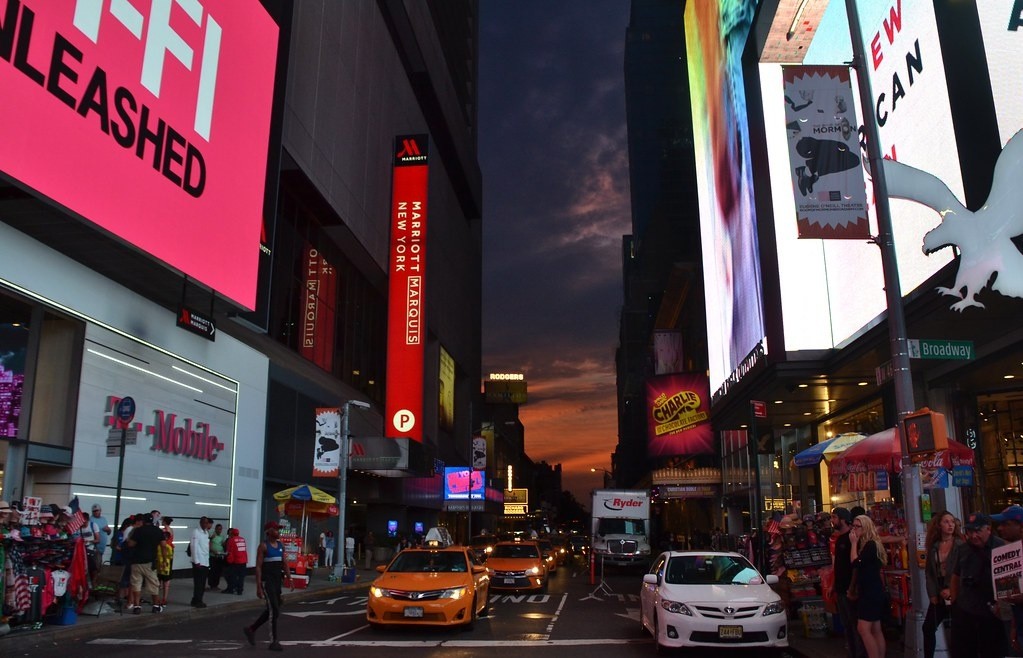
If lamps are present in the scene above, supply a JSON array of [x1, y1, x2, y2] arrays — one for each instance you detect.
[[992, 404, 996, 412], [980, 409, 985, 415], [983, 416, 988, 422]]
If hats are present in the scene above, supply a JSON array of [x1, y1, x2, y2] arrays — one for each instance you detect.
[[92, 504, 101, 512], [264, 521, 284, 531], [0, 494, 76, 569], [142, 514, 154, 523], [962, 512, 988, 530], [769, 506, 835, 576], [988, 505, 1023, 523], [231, 529, 239, 535]]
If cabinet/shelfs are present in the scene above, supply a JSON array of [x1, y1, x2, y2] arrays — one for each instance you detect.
[[770, 546, 832, 620]]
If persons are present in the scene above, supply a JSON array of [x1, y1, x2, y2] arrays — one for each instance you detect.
[[243, 521, 294, 650], [0, 500, 174, 615], [188, 516, 247, 608], [832, 504, 888, 658], [318, 528, 463, 572], [922, 504, 1023, 658]]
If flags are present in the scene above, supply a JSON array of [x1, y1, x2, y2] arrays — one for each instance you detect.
[[9, 544, 31, 612], [63, 498, 85, 534]]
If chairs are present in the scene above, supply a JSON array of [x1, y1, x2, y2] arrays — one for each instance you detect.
[[722, 563, 743, 583], [82, 560, 127, 619]]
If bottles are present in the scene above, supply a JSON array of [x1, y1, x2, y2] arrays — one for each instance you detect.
[[987, 601, 1005, 619], [943, 591, 952, 612], [872, 501, 907, 537], [893, 539, 909, 569], [289, 579, 294, 595], [888, 573, 913, 617], [1011, 640, 1016, 656]]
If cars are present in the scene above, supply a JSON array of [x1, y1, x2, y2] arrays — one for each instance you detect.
[[464, 534, 499, 559], [483, 531, 549, 594], [538, 535, 592, 574], [368, 528, 491, 630], [640, 549, 788, 657]]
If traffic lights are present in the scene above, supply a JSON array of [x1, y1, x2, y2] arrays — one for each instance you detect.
[[899, 410, 947, 461]]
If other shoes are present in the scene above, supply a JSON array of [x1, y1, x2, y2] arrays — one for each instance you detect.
[[133, 606, 142, 614], [211, 586, 220, 591], [193, 600, 206, 608], [270, 642, 282, 650], [151, 605, 162, 613], [243, 627, 255, 645], [222, 588, 234, 594]]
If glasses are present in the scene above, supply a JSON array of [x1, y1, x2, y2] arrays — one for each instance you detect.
[[851, 524, 861, 529]]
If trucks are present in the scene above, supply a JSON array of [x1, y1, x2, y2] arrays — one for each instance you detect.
[[591, 489, 652, 572]]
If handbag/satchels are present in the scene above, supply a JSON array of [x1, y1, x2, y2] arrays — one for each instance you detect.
[[186, 543, 192, 557]]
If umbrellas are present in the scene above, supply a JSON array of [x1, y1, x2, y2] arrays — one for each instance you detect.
[[789, 426, 975, 505], [273, 485, 339, 549]]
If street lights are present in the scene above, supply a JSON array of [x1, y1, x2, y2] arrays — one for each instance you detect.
[[469, 420, 516, 544], [334, 400, 372, 578]]
